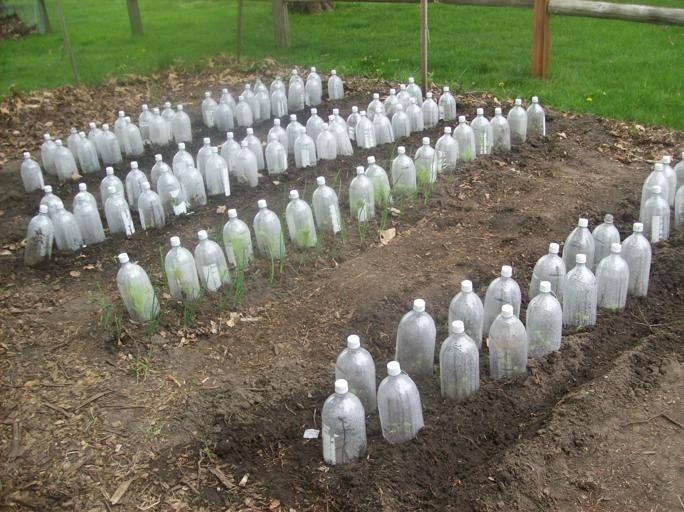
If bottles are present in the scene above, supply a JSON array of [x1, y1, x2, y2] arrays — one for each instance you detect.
[[392, 146, 416, 195], [222, 209, 253, 269], [349, 166, 375, 222], [674, 185, 684, 231], [526, 97, 545, 137], [20, 121, 104, 266], [594, 243, 629, 309], [219, 121, 283, 197], [414, 137, 437, 184], [490, 108, 511, 155], [388, 120, 431, 141], [193, 230, 230, 293], [469, 108, 491, 156], [525, 281, 562, 357], [507, 99, 527, 145], [488, 304, 527, 380], [640, 185, 670, 243], [660, 156, 677, 207], [528, 242, 566, 301], [591, 214, 620, 275], [104, 114, 162, 239], [253, 199, 285, 260], [438, 320, 479, 401], [395, 299, 436, 380], [164, 236, 200, 301], [116, 253, 160, 323], [483, 265, 521, 336], [349, 123, 388, 156], [116, 66, 457, 122], [673, 152, 684, 189], [435, 127, 457, 174], [447, 279, 484, 351], [162, 122, 221, 219], [334, 334, 377, 416], [620, 222, 652, 297], [320, 378, 367, 466], [562, 218, 594, 275], [364, 156, 392, 208], [562, 254, 597, 327], [283, 123, 348, 172], [312, 176, 341, 235], [641, 163, 670, 211], [452, 116, 475, 162], [285, 190, 316, 249], [376, 361, 424, 444]]
